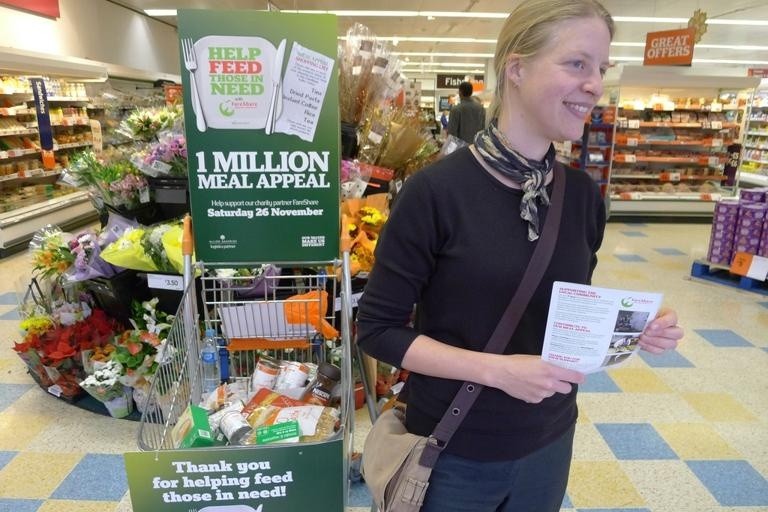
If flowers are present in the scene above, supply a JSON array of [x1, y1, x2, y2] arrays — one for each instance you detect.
[[27, 210, 183, 296], [56, 99, 182, 209], [342, 22, 444, 405], [15, 296, 182, 425]]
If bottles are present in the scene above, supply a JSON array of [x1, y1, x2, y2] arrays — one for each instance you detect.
[[198, 329, 230, 395], [307, 362, 342, 406], [746, 148, 760, 160]]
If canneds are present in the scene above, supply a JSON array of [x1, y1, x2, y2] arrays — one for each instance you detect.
[[219, 410, 253, 444], [250, 354, 318, 393]]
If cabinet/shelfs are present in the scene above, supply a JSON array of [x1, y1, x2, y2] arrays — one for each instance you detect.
[[0, 93, 162, 211], [565, 67, 768, 224]]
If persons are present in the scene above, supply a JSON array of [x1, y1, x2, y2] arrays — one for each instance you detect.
[[444, 82, 487, 143], [356, 1, 684, 512]]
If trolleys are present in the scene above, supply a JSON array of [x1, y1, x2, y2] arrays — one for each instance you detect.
[[130, 214, 384, 511]]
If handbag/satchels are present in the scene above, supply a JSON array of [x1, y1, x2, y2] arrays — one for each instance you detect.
[[358, 408, 442, 512]]
